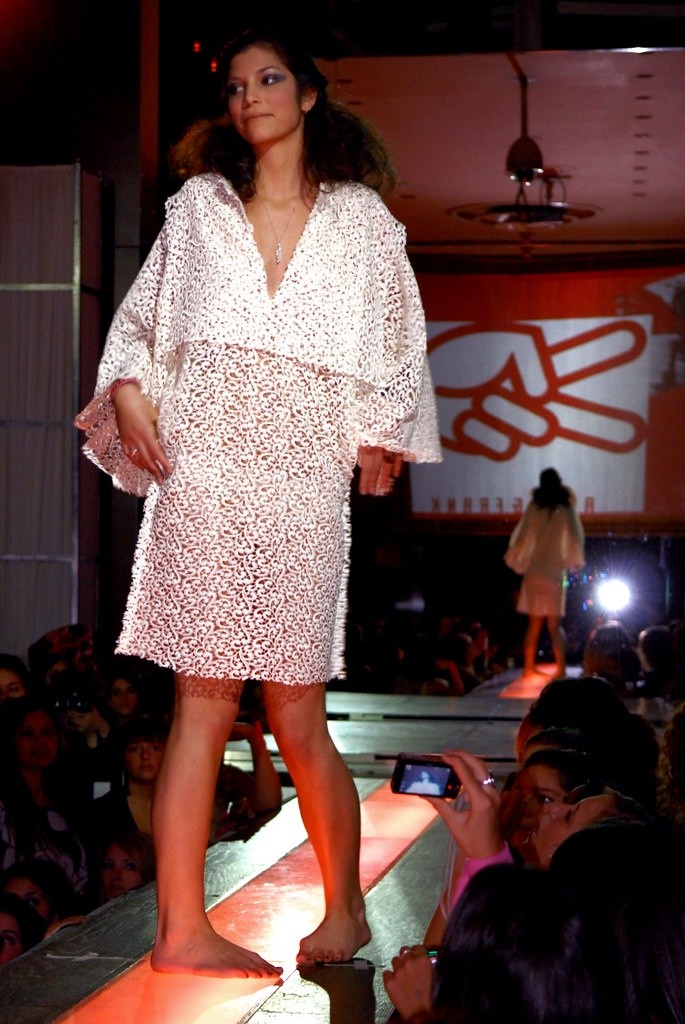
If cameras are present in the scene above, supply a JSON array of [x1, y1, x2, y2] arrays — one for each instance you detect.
[[428, 950, 440, 965], [389, 752, 462, 800]]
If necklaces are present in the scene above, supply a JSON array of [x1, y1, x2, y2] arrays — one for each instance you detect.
[[260, 198, 297, 264]]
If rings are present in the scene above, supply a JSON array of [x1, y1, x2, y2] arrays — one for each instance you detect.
[[481, 778, 495, 785], [132, 449, 137, 457], [402, 949, 413, 957]]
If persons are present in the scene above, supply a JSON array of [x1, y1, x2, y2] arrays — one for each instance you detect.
[[72, 34, 440, 978], [346, 604, 502, 700], [383, 611, 685, 1024], [504, 468, 584, 682], [406, 770, 440, 794], [0, 623, 283, 966]]
